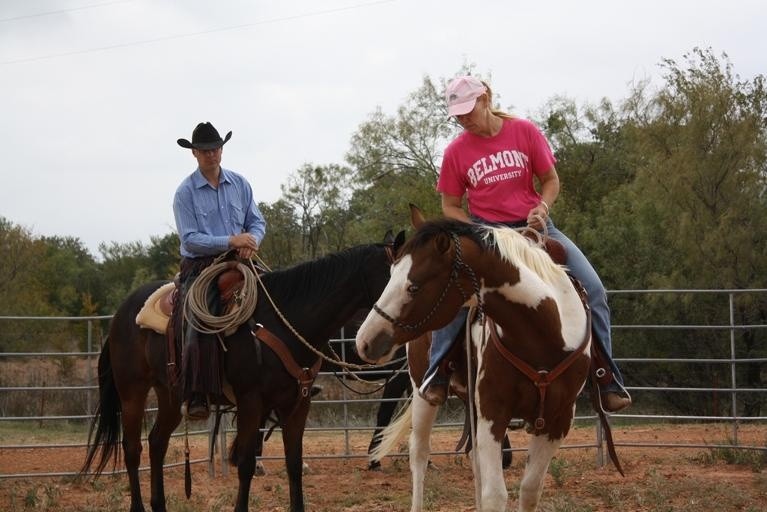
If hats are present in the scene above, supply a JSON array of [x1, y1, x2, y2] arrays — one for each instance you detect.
[[445, 76, 487, 121], [177, 122, 232, 151]]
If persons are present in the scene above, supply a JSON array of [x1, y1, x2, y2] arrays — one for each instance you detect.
[[173, 122, 324, 418], [422, 76, 631, 411]]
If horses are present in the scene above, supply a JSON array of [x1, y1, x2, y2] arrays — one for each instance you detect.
[[355, 203, 593, 512], [79, 230, 406, 512], [228, 309, 511, 476]]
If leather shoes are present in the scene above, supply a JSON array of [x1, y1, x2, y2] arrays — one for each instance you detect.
[[185, 399, 208, 421], [593, 392, 630, 413], [422, 380, 446, 405]]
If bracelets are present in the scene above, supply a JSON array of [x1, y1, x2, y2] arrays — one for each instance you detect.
[[540, 201, 549, 215]]
